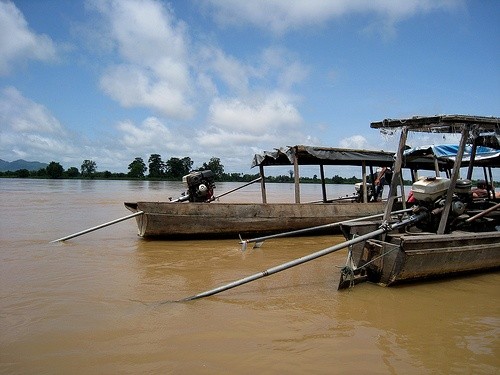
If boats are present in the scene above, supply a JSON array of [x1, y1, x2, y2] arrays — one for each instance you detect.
[[123, 141, 461, 241], [337, 112, 500, 291]]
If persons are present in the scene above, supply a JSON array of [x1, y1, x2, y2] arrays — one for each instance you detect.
[[374, 166, 387, 202]]
